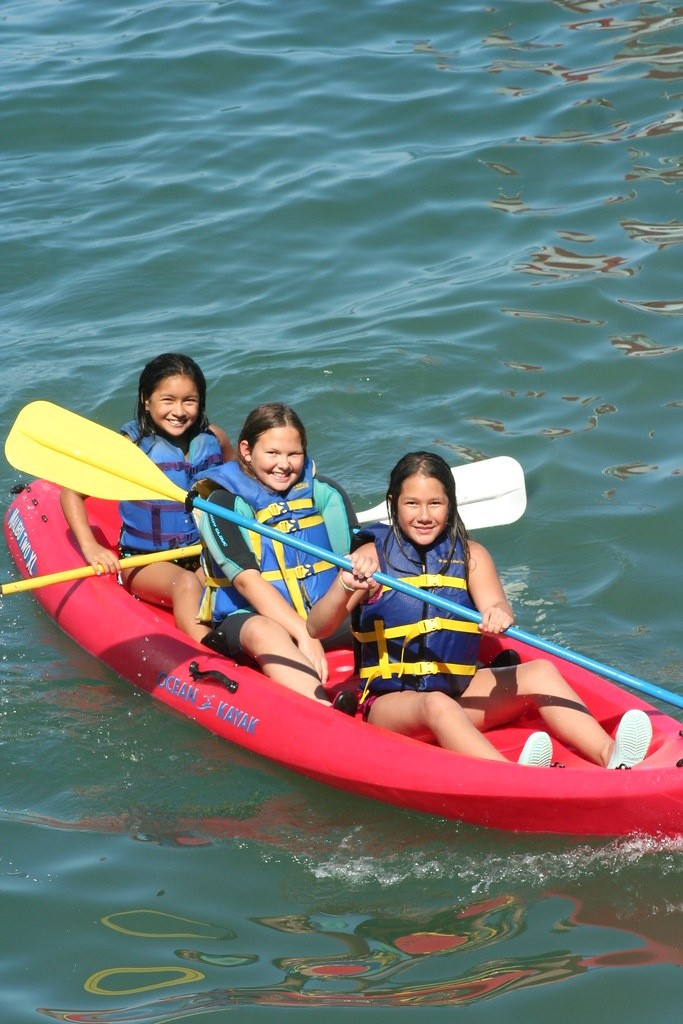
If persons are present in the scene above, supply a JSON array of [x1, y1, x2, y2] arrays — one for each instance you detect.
[[306, 450, 652, 769], [184, 403, 378, 718], [60, 354, 237, 645]]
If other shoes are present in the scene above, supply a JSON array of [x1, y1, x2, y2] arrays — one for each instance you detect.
[[606, 709, 653, 769], [518, 731, 554, 768], [329, 690, 358, 718], [485, 649, 521, 668]]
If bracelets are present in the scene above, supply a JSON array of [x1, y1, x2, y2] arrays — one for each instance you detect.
[[340, 574, 356, 592]]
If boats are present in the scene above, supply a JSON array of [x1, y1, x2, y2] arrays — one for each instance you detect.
[[4, 477, 682, 838]]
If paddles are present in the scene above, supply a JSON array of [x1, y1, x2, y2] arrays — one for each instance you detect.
[[1, 453, 530, 599], [1, 395, 683, 712]]
